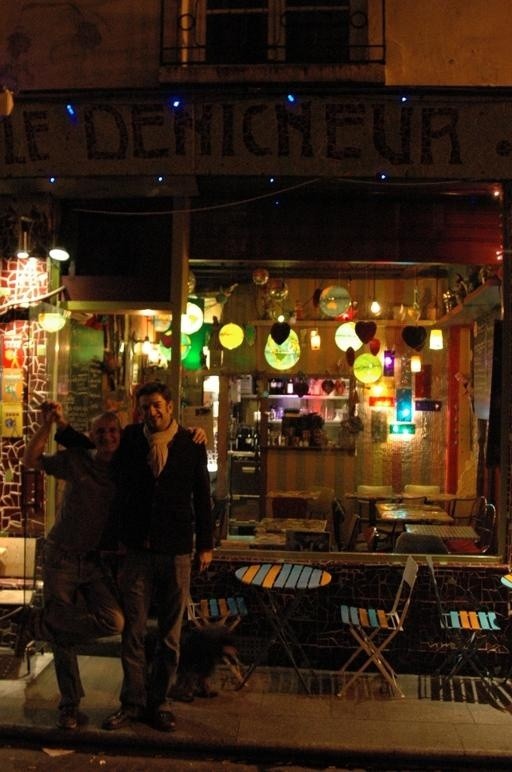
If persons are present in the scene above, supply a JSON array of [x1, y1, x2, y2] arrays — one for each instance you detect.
[[40, 380, 216, 730], [12, 403, 208, 730]]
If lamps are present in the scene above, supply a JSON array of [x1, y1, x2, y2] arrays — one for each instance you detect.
[[16, 241, 445, 441]]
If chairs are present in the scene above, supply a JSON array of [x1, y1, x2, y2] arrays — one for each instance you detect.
[[0, 537, 37, 658], [227, 485, 497, 554], [188, 597, 264, 687], [425, 555, 501, 703], [336, 554, 419, 699]]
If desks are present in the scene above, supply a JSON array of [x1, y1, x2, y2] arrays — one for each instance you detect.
[[501, 572, 512, 596], [234, 564, 333, 695]]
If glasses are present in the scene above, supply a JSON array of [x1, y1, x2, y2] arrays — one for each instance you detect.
[[96, 428, 119, 434]]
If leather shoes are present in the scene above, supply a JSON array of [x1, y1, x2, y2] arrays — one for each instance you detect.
[[56, 705, 78, 728], [102, 710, 133, 730], [148, 706, 177, 732], [15, 609, 32, 658]]
